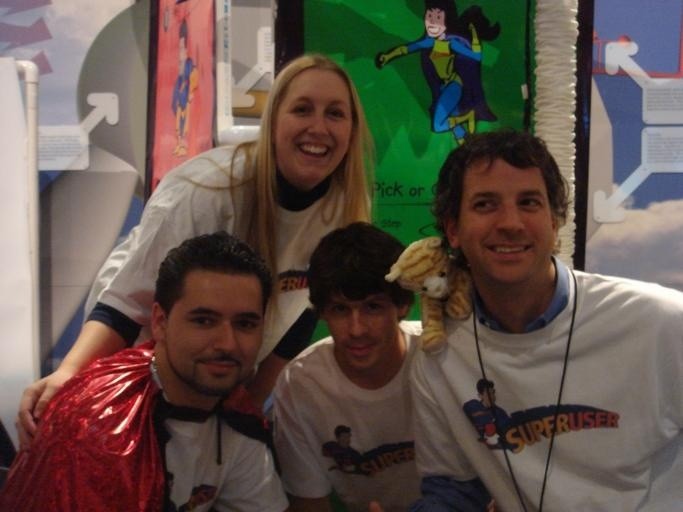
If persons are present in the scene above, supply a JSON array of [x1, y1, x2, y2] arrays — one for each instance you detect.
[[376, 2, 503, 145], [414, 130, 683, 508], [272, 223, 424, 507], [468, 378, 520, 454], [15, 54, 370, 446], [1, 232, 290, 508], [315, 418, 372, 479]]
